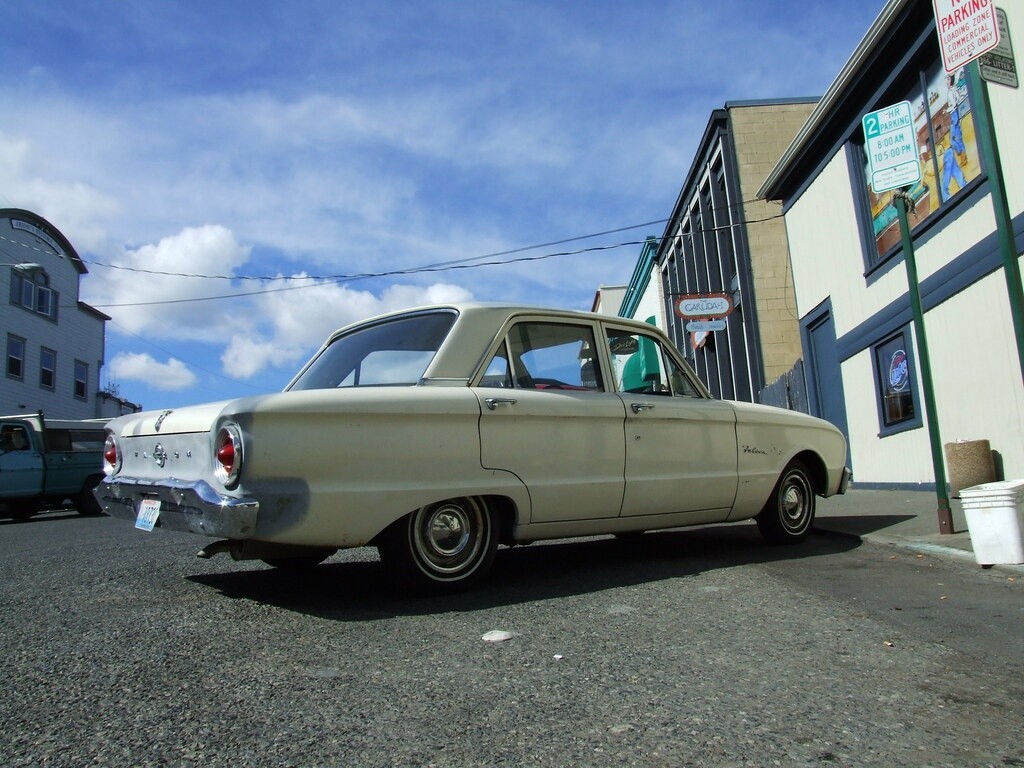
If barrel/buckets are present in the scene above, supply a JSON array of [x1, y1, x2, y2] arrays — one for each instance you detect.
[[960, 478, 1024, 565]]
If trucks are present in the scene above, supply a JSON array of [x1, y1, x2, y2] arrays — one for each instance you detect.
[[0, 408, 107, 516]]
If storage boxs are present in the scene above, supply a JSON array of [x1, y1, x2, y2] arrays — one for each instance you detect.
[[959, 479, 1024, 565]]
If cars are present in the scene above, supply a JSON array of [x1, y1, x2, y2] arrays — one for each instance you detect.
[[91, 303, 856, 595]]
